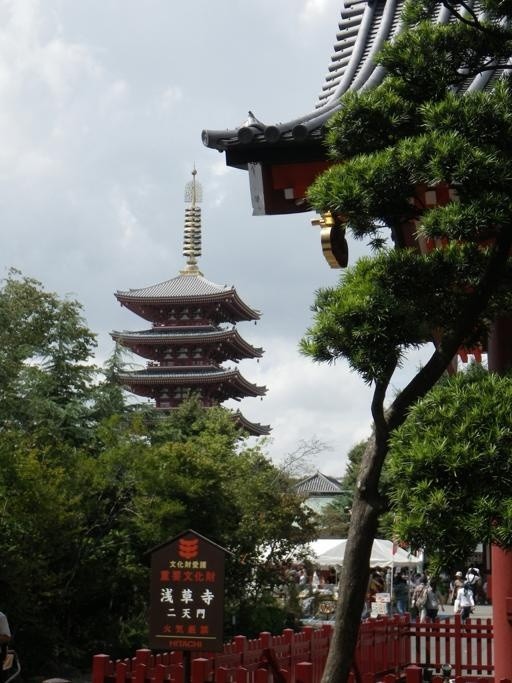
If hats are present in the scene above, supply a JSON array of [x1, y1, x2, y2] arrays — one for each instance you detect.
[[454, 570, 463, 577]]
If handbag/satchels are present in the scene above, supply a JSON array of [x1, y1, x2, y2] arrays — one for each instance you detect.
[[415, 587, 429, 609]]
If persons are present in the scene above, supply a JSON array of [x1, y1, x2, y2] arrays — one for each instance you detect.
[[279, 560, 493, 640], [0, 611, 12, 654]]
